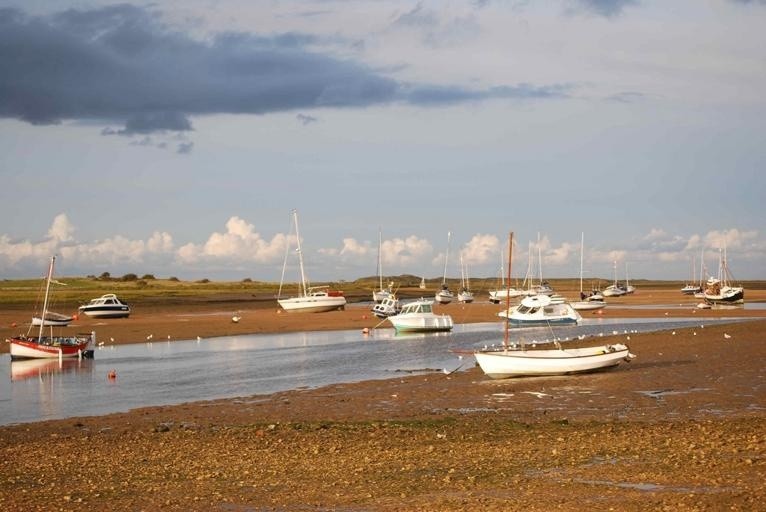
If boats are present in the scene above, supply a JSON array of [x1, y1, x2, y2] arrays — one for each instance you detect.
[[78, 294, 132, 319], [32, 316, 75, 327]]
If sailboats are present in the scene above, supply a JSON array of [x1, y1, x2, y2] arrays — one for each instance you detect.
[[369, 227, 636, 334], [682, 241, 746, 306], [472, 231, 638, 379], [6, 255, 97, 362], [277, 209, 346, 315]]
